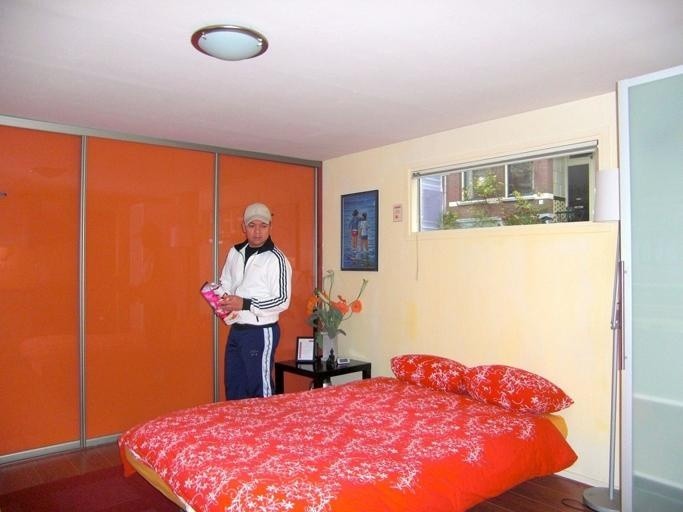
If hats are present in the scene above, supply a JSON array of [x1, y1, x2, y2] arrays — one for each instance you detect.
[[243, 203, 271, 226]]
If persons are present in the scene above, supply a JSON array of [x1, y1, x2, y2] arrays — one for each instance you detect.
[[357, 213, 369, 252], [348, 209, 367, 249], [209, 202, 292, 400]]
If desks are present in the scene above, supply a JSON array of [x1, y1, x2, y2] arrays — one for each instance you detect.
[[273, 355, 372, 394]]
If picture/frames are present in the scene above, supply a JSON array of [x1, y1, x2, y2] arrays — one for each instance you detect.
[[340, 189, 379, 272], [295, 336, 315, 364]]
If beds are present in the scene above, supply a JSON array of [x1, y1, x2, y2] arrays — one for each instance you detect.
[[117, 376, 568, 512]]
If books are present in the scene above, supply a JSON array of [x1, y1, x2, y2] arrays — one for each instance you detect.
[[199, 280, 241, 326]]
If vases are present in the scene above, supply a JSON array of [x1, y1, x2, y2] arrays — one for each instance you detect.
[[320, 330, 335, 362]]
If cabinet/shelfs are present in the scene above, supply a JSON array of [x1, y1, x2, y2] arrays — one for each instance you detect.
[[218, 144, 322, 399], [2, 114, 82, 462], [83, 126, 217, 464]]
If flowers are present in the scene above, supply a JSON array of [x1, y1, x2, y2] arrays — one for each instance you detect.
[[305, 269, 369, 348]]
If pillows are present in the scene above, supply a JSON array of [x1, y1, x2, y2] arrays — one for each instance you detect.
[[390, 353, 470, 395], [452, 363, 575, 415]]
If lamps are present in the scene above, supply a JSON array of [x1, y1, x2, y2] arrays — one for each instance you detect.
[[580, 166, 625, 511], [191, 23, 269, 62]]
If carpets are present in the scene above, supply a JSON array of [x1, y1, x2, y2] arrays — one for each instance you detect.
[[0, 462, 184, 512]]
